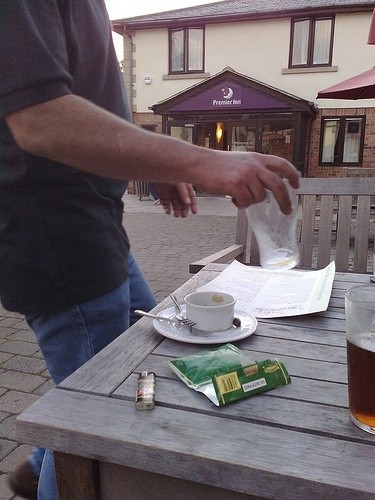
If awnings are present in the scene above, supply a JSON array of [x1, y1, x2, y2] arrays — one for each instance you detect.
[[316, 66, 375, 100]]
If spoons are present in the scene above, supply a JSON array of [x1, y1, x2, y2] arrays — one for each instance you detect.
[[170, 294, 186, 321]]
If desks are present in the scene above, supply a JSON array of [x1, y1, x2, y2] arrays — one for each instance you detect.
[[16, 263, 375, 500]]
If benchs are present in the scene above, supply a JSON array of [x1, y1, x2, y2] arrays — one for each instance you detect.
[[189, 176, 375, 273]]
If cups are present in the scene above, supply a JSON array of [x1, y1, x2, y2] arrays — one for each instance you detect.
[[184, 291, 237, 332], [245, 179, 300, 270], [344, 284, 375, 436]]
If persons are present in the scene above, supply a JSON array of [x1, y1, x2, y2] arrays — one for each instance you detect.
[[0, 0, 300, 500]]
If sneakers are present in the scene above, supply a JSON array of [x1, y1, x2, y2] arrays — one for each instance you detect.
[[9, 461, 40, 500]]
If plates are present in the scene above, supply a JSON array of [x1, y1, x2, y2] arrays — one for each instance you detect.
[[153, 305, 257, 344]]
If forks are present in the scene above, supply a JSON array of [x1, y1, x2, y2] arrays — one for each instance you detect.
[[134, 310, 197, 326]]
